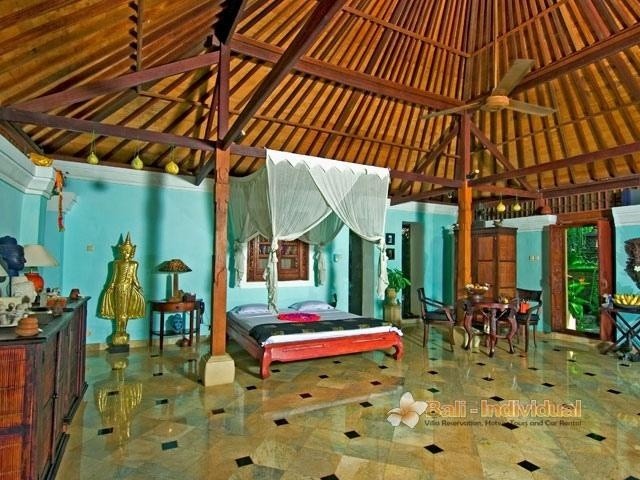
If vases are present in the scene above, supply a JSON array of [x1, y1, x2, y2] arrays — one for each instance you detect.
[[469, 293, 483, 303]]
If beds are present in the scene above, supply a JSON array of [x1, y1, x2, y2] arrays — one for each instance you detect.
[[465, 298, 519, 357], [227, 300, 404, 379]]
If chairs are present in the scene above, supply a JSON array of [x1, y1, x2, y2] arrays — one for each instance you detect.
[[496, 288, 543, 351], [419, 288, 457, 352]]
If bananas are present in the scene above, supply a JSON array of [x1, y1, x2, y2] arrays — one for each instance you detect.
[[612, 294, 640, 305]]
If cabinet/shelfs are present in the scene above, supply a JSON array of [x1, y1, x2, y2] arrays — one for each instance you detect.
[[150, 300, 201, 348], [0, 312, 70, 480], [454, 228, 518, 327], [64, 296, 89, 427]]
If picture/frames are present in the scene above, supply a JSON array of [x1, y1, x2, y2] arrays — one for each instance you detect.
[[386, 249, 394, 260], [386, 233, 395, 245]]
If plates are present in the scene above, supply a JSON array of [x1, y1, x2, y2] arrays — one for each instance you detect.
[[615, 304, 640, 311], [469, 289, 487, 299]]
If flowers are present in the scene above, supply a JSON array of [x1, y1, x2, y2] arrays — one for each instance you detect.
[[465, 283, 489, 295]]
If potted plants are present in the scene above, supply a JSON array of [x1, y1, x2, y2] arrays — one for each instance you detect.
[[385, 269, 412, 301]]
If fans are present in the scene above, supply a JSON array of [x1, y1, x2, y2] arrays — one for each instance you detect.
[[420, 0, 557, 120]]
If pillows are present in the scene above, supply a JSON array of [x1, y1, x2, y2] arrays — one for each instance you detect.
[[289, 300, 334, 311], [159, 260, 192, 302], [231, 303, 272, 314]]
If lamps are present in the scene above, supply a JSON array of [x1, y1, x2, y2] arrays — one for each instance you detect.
[[24, 244, 58, 291]]
